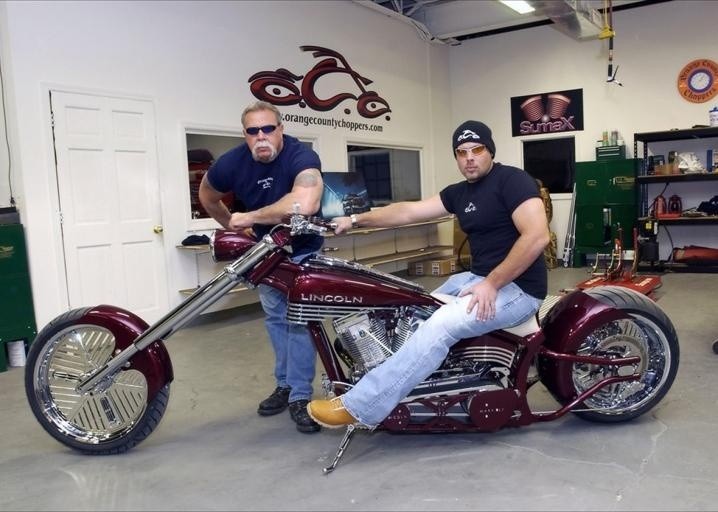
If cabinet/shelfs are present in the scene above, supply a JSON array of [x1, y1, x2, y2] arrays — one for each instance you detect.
[[0, 223, 38, 372], [633, 127, 718, 273], [572, 155, 645, 267], [175, 216, 457, 297]]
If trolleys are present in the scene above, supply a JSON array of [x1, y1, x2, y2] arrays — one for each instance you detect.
[[567, 216, 665, 305]]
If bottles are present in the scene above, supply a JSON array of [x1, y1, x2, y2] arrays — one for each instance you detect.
[[654, 195, 682, 216], [602, 127, 618, 146]]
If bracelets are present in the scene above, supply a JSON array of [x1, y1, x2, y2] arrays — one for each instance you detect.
[[350, 214, 359, 229]]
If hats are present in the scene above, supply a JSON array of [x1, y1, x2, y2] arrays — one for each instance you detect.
[[451, 119, 496, 160]]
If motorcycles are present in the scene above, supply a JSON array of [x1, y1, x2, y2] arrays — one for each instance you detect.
[[16, 199, 684, 479]]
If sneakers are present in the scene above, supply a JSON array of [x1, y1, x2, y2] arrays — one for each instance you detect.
[[257, 386, 291, 417], [287, 398, 321, 435], [306, 394, 373, 432]]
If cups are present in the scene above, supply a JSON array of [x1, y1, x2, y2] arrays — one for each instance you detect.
[[616, 139, 625, 146], [660, 164, 674, 175]]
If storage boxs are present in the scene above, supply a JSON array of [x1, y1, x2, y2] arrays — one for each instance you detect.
[[408, 253, 472, 276]]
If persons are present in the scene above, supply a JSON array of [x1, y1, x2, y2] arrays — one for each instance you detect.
[[307, 119, 550, 432], [199, 102, 325, 433]]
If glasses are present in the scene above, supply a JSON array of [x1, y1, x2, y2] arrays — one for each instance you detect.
[[245, 124, 279, 134], [453, 144, 487, 157]]
